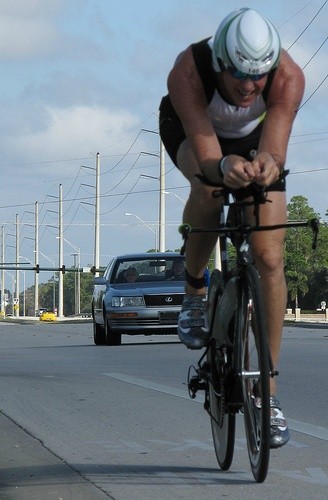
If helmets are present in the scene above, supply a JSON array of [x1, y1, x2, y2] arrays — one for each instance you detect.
[[212, 7, 280, 75]]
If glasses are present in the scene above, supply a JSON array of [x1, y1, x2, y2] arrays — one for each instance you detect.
[[228, 67, 267, 81]]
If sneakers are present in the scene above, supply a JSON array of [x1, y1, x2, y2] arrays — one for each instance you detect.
[[177, 295, 210, 348], [252, 384, 290, 448]]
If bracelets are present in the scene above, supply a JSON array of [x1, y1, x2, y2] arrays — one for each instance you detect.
[[217, 155, 227, 178]]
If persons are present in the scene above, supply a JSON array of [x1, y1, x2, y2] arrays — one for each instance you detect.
[[124, 267, 139, 282], [166, 259, 187, 280], [159, 7, 306, 449]]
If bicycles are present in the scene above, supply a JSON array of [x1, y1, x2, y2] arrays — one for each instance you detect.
[[179, 167, 319, 484]]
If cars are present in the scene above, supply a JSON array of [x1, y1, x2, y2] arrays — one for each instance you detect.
[[38, 308, 47, 317], [39, 312, 56, 321], [91, 252, 210, 346]]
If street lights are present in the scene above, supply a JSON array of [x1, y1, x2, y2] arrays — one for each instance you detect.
[[55, 236, 81, 314], [124, 213, 158, 273], [32, 250, 55, 314]]
